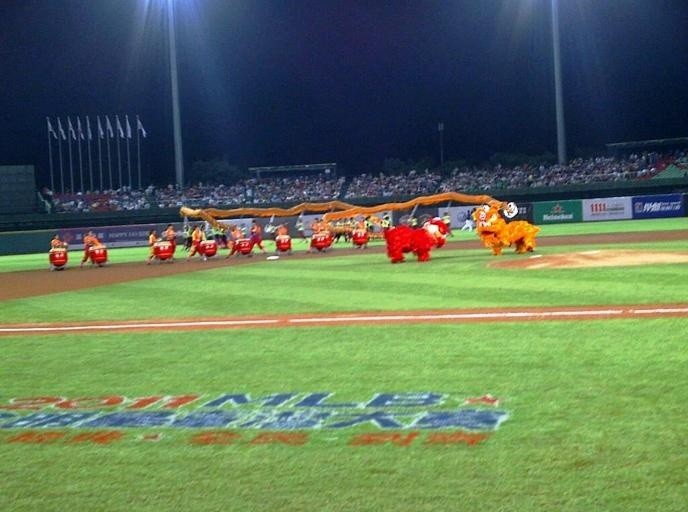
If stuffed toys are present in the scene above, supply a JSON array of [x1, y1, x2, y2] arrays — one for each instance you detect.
[[472, 205, 540, 256], [383, 217, 451, 264]]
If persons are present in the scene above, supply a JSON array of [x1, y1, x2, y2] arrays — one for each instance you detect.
[[45, 148, 688, 216], [398, 207, 476, 238], [50, 231, 103, 267], [148, 212, 390, 262]]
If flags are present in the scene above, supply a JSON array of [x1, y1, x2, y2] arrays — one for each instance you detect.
[[86, 116, 92, 140], [138, 119, 146, 138], [97, 116, 104, 139], [77, 117, 84, 140], [116, 114, 124, 138], [126, 118, 131, 138], [106, 115, 113, 138], [58, 119, 66, 140], [48, 120, 57, 139], [67, 116, 76, 141]]
